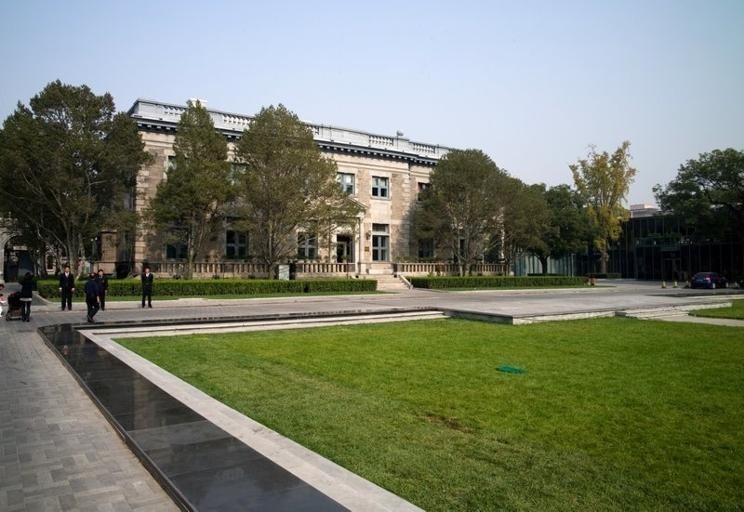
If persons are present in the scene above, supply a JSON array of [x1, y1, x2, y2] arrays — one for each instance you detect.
[[83, 271, 101, 323], [19, 272, 33, 322], [0, 281, 4, 291], [0, 294, 7, 320], [140, 266, 153, 309], [55, 265, 74, 311], [94, 268, 108, 311]]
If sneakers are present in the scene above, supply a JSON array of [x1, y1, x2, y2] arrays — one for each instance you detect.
[[87, 314, 93, 322]]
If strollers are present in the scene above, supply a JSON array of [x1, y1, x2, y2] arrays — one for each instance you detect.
[[6, 292, 23, 321]]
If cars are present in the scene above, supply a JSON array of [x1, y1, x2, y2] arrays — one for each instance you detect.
[[692, 272, 726, 289]]
